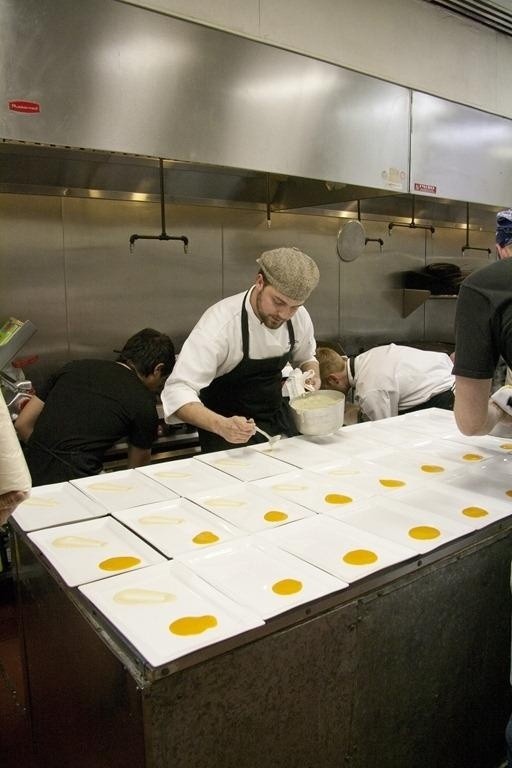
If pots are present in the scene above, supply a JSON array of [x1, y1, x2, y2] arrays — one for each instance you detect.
[[289, 390, 344, 436]]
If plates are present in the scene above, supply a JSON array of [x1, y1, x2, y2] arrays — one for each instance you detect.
[[112, 497, 248, 560], [248, 430, 344, 470], [9, 482, 109, 534], [184, 481, 319, 535], [136, 453, 249, 496], [77, 558, 267, 667], [68, 468, 179, 512], [253, 513, 420, 585], [250, 466, 367, 515], [195, 439, 302, 483], [305, 406, 512, 531], [325, 494, 476, 555], [26, 514, 164, 588], [175, 534, 349, 622]]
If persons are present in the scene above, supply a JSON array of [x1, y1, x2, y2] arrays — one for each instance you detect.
[[160, 245, 323, 454], [314, 340, 457, 424], [449, 207, 511, 437], [12, 327, 178, 489]]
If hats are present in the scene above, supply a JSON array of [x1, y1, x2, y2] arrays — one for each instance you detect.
[[256, 246, 320, 301], [495, 209, 512, 230]]
